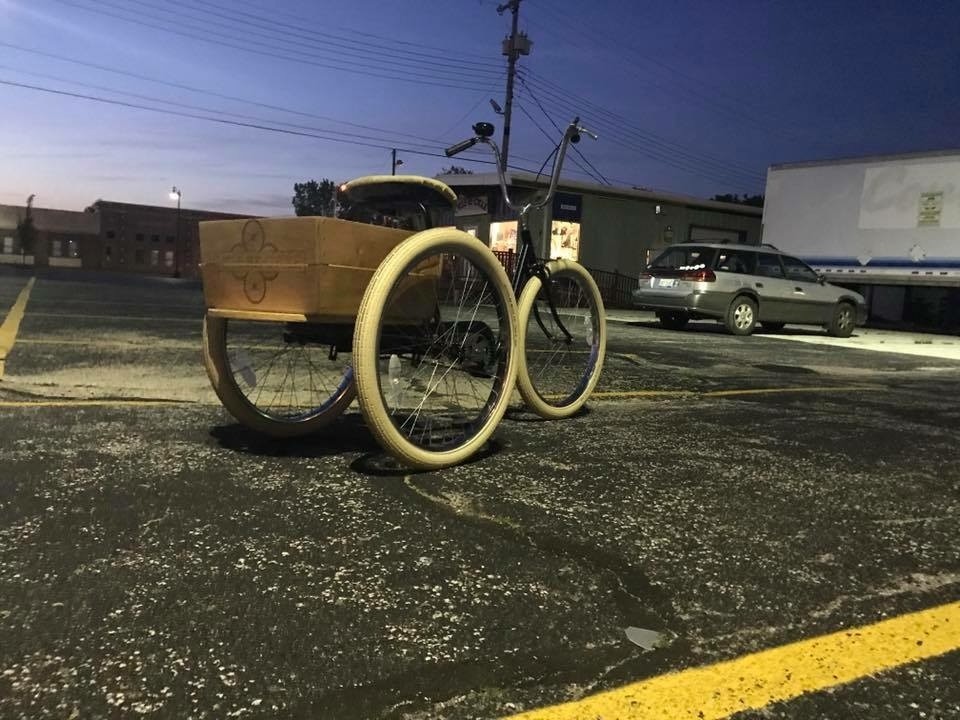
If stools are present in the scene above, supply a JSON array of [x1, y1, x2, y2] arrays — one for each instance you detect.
[[341, 175, 457, 229]]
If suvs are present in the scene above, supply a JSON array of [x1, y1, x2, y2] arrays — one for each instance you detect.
[[628, 240, 868, 338]]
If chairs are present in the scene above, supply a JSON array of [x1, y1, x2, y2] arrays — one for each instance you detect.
[[735, 263, 749, 273], [758, 265, 773, 276], [691, 253, 722, 268]]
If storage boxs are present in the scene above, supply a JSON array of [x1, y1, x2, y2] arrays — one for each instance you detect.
[[198, 218, 438, 319]]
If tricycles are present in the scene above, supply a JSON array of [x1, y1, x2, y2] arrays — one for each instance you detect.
[[197, 116, 608, 474]]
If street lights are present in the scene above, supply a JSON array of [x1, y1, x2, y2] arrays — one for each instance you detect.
[[168, 186, 182, 279], [391, 148, 404, 176], [488, 99, 514, 269]]
